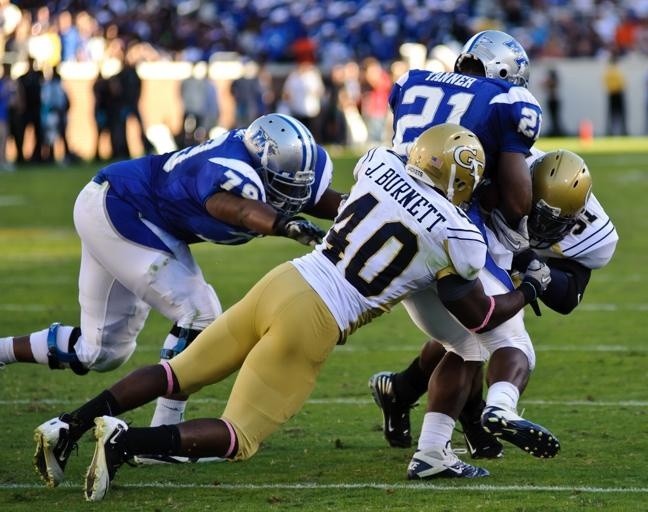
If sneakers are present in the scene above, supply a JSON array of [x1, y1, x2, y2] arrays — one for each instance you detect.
[[83, 415, 130, 501], [461, 402, 504, 461], [480, 406, 560, 458], [134, 452, 225, 465], [368, 370, 412, 447], [407, 438, 490, 480], [32, 412, 77, 488]]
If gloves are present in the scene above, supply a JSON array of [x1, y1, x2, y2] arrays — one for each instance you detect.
[[272, 213, 325, 245], [516, 258, 552, 303], [491, 209, 533, 266]]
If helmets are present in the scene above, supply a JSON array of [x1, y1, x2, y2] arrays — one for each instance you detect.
[[454, 29, 530, 88], [244, 112, 317, 216], [527, 149, 593, 250], [406, 122, 486, 205]]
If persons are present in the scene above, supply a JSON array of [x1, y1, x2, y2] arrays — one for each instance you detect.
[[32, 121, 552, 502], [368, 29, 561, 482], [0, 114, 346, 465], [1, 1, 647, 168], [369, 145, 620, 461]]
